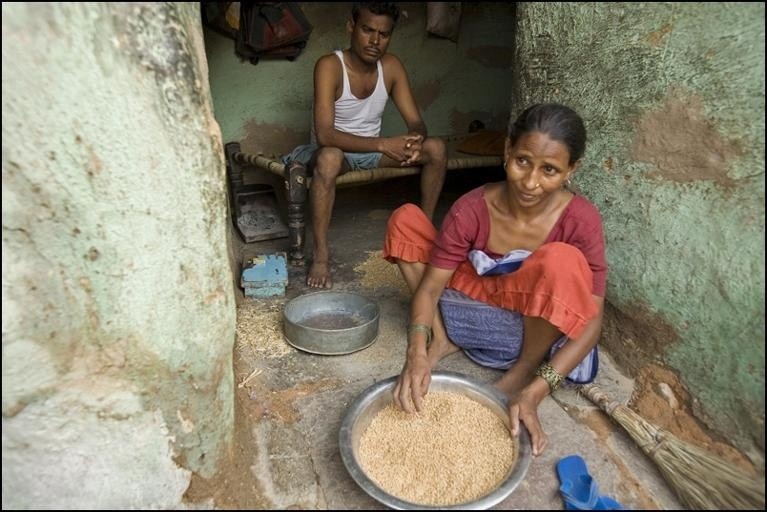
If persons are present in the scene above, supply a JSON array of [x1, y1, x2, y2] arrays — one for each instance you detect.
[[381, 103, 608, 457], [306, 2, 448, 289]]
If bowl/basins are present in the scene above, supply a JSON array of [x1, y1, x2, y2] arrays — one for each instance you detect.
[[336, 370, 536, 510]]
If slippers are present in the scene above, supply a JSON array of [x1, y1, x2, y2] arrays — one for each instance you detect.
[[555, 455, 608, 511]]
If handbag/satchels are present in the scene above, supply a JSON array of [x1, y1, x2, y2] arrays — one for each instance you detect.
[[235, 2, 314, 65], [425, 0, 462, 43]]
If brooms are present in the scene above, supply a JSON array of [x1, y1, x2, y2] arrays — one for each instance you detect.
[[576, 377, 765, 511]]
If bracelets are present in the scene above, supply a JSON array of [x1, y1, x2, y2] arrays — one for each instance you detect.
[[536, 362, 561, 395], [408, 324, 432, 350]]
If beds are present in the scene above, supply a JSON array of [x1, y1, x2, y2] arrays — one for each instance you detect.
[[224, 139, 504, 265]]
